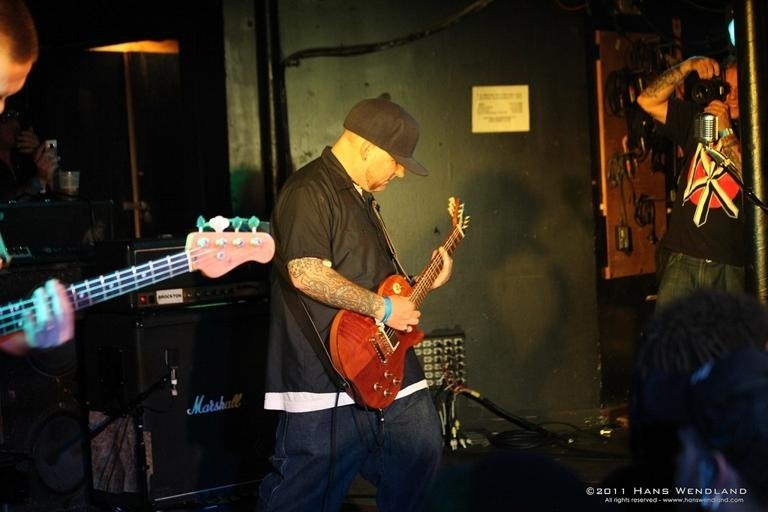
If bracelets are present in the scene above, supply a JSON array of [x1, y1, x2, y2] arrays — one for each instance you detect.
[[380, 296, 392, 323]]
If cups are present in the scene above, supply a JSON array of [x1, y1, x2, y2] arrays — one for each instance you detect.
[[57, 166, 80, 202]]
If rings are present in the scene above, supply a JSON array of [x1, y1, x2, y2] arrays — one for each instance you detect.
[[404, 326, 408, 333]]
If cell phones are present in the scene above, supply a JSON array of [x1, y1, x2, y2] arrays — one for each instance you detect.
[[45, 139, 58, 157]]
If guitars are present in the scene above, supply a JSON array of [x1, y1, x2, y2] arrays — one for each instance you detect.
[[0, 217, 275, 338], [330, 196, 471, 409]]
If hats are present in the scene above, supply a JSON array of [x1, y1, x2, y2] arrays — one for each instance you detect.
[[342, 97, 429, 176]]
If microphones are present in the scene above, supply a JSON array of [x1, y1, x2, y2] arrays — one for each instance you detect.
[[692, 111, 718, 144]]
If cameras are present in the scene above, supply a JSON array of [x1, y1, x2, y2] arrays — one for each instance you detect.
[[692, 69, 731, 106]]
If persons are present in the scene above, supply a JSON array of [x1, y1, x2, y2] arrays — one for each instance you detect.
[[0, 0, 77, 358], [586, 286, 768, 510], [640, 343, 768, 510], [257, 98, 452, 512], [0, 104, 54, 200], [634, 53, 765, 312]]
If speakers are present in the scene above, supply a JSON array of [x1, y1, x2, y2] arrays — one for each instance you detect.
[[128, 302, 267, 504], [0, 260, 134, 512]]
